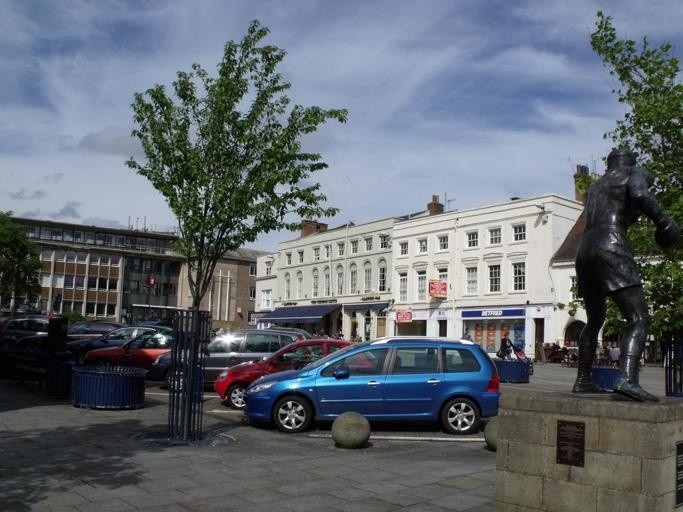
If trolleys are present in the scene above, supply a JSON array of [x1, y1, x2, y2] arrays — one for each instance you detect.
[[510, 349, 534, 376]]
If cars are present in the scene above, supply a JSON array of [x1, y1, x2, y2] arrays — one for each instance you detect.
[[243, 336, 500, 433]]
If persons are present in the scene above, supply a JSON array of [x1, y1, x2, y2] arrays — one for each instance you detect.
[[571, 148, 681, 401], [461, 328, 473, 342], [500, 331, 520, 360]]
